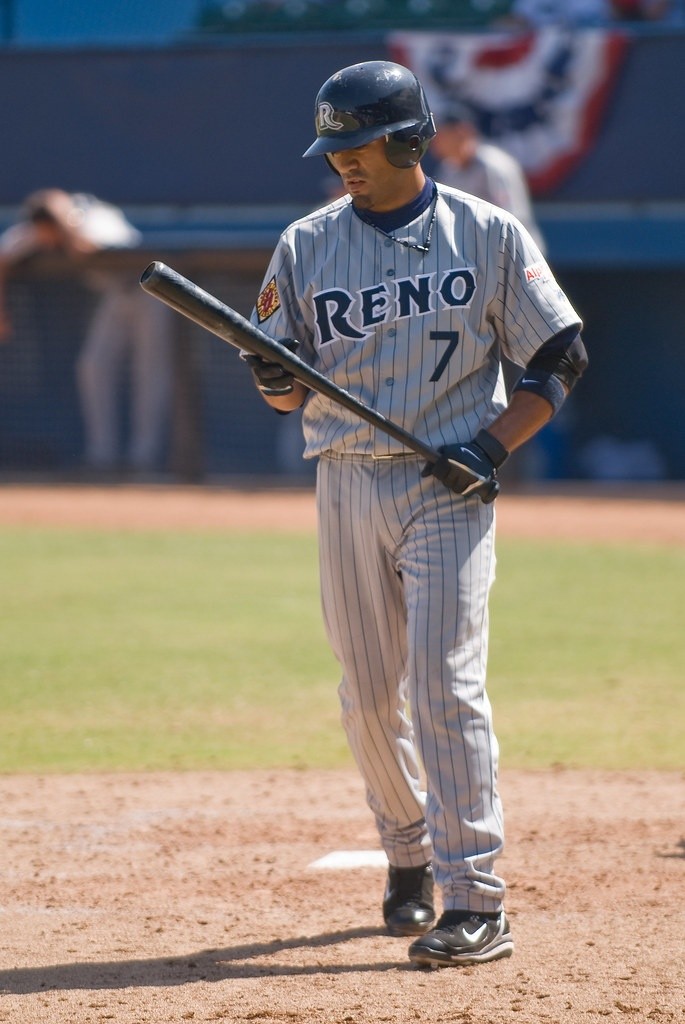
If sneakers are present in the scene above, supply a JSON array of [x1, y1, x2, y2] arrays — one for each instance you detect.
[[408, 910, 514, 969], [383, 862, 435, 935]]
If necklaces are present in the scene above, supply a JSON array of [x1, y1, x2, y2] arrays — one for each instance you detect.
[[358, 177, 440, 255]]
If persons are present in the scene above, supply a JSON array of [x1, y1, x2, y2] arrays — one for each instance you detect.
[[430, 101, 546, 257], [239, 60, 589, 966], [0, 188, 170, 480]]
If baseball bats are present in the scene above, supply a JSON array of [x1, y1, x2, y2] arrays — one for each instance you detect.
[[139, 260, 502, 506]]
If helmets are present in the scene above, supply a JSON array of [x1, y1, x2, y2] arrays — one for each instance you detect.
[[301, 60, 437, 176]]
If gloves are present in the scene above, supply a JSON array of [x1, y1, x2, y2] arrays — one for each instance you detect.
[[243, 338, 300, 396], [420, 428, 510, 498]]
